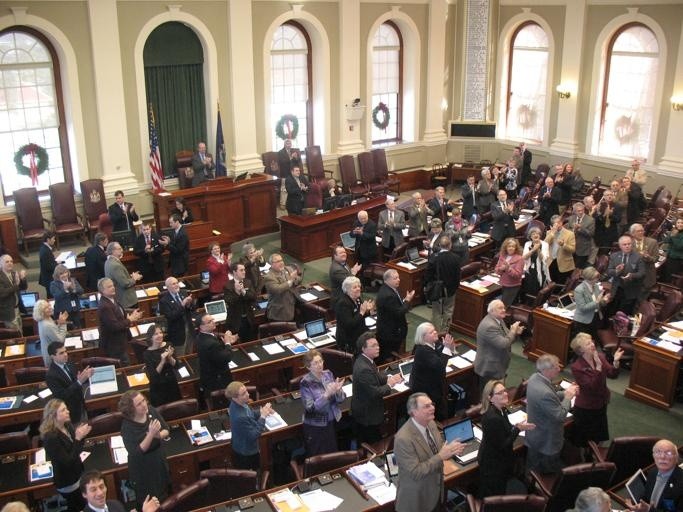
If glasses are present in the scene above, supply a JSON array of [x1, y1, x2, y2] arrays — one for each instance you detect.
[[492, 387, 510, 396]]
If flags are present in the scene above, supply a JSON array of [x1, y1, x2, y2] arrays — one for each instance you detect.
[[215, 110, 228, 177], [145, 108, 169, 193]]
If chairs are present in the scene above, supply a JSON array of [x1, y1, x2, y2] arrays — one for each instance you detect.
[[338, 149, 401, 197], [50, 183, 87, 251], [464, 436, 670, 512], [177, 150, 199, 189], [15, 187, 54, 256], [432, 164, 448, 188], [304, 146, 337, 191], [81, 179, 109, 244]]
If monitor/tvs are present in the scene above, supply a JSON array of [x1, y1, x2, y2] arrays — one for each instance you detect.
[[323, 194, 353, 212], [234, 172, 248, 181], [112, 227, 175, 251]]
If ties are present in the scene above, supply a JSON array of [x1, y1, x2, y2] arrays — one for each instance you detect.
[[63, 363, 73, 380], [114, 299, 125, 320], [389, 210, 393, 225], [425, 427, 439, 456], [622, 253, 628, 271]]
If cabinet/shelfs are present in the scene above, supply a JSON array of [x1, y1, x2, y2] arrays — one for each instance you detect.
[[2, 213, 21, 263]]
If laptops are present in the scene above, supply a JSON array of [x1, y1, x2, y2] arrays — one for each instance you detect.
[[19, 292, 39, 318], [407, 246, 428, 265], [398, 358, 415, 382], [444, 418, 482, 465], [204, 299, 228, 323], [304, 318, 336, 348], [385, 450, 399, 488], [559, 293, 576, 312], [340, 231, 356, 252], [626, 469, 648, 504], [200, 271, 210, 284], [88, 364, 119, 396]]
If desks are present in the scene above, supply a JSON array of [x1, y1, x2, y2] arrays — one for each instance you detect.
[[330, 199, 681, 414], [278, 192, 399, 261], [1, 221, 526, 512], [449, 163, 502, 187], [155, 171, 280, 239]]
